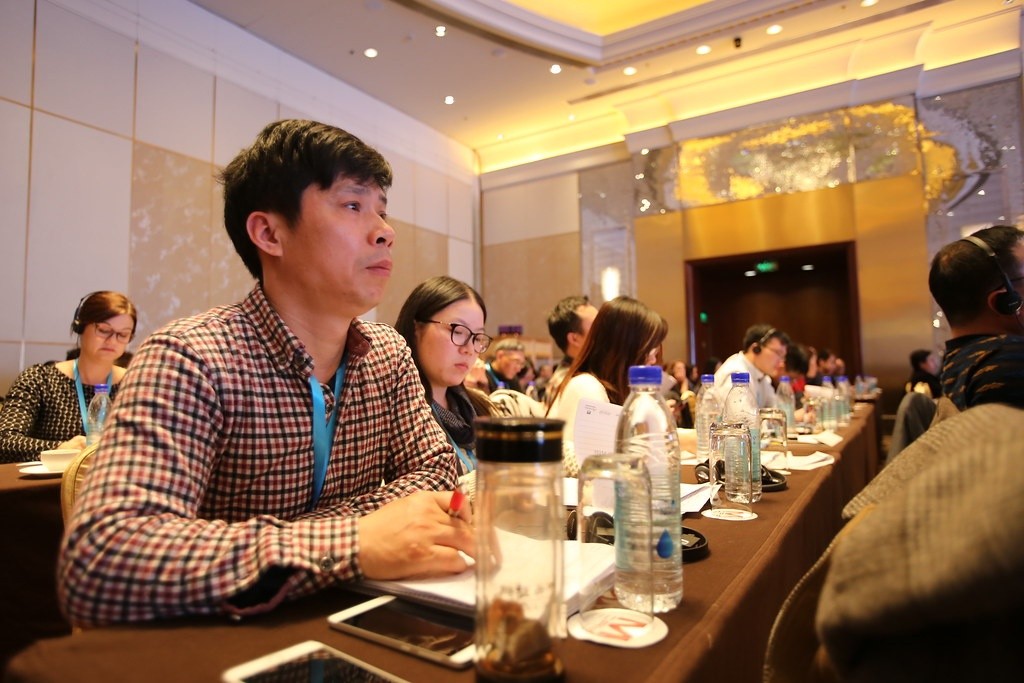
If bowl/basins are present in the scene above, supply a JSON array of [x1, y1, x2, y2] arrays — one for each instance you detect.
[[40, 449, 82, 472]]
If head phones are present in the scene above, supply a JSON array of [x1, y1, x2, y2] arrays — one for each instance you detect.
[[695, 459, 787, 491], [959, 236, 1021, 315], [753, 328, 776, 353], [73, 292, 97, 334], [567, 509, 708, 561]]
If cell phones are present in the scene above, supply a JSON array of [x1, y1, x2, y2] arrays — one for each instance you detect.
[[328, 595, 492, 668], [222, 639, 412, 683]]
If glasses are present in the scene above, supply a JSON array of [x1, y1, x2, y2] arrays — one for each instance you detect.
[[91, 322, 135, 344], [415, 319, 494, 354]]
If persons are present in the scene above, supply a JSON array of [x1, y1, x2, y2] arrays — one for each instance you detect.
[[462, 338, 559, 403], [661, 357, 722, 430], [928, 225, 1024, 409], [546, 294, 599, 408], [546, 295, 698, 481], [692, 324, 807, 433], [768, 344, 846, 410], [909, 350, 942, 398], [394, 276, 501, 513], [51, 117, 497, 630], [0, 292, 137, 464]]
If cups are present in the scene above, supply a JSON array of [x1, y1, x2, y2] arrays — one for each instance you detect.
[[708, 421, 753, 519], [576, 456, 656, 630], [803, 396, 824, 432], [759, 408, 788, 470]]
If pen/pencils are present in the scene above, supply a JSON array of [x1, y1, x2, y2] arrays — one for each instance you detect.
[[448, 482, 465, 515]]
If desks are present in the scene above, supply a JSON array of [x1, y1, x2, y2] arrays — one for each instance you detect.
[[3, 403, 879, 683], [0, 461, 69, 683]]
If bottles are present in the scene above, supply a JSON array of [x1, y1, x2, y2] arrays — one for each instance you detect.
[[696, 374, 724, 464], [496, 382, 506, 389], [774, 375, 795, 433], [615, 363, 684, 613], [526, 381, 539, 402], [838, 374, 878, 428], [469, 416, 569, 683], [725, 372, 762, 502], [821, 376, 838, 431], [85, 383, 113, 449]]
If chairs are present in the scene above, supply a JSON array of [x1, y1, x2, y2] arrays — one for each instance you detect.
[[886, 391, 936, 464]]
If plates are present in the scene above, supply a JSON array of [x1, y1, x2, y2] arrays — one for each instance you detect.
[[19, 465, 64, 476]]
